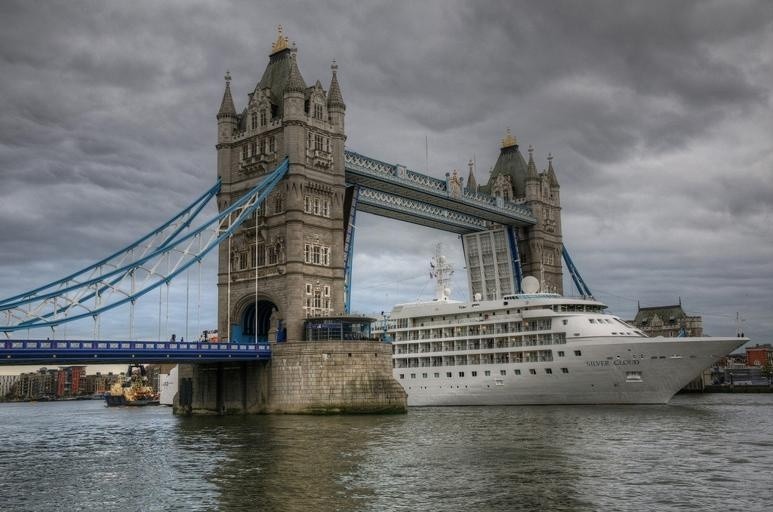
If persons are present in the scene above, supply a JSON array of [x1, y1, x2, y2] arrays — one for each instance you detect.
[[170, 334, 176, 341]]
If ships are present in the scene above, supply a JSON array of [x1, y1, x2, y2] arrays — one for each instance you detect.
[[103, 364, 160, 406], [159, 240, 751, 406]]
